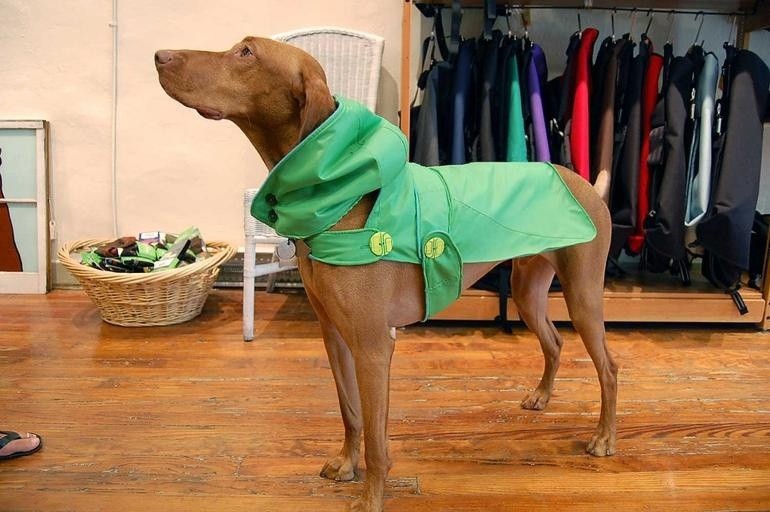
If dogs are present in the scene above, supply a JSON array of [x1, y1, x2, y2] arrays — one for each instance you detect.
[[154, 36, 619, 512]]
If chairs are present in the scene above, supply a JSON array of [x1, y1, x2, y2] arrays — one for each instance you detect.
[[238, 27, 392, 342]]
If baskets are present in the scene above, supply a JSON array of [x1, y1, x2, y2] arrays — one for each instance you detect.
[[56, 237, 236, 328]]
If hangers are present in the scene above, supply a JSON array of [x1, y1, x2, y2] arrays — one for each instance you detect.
[[443, 4, 742, 59]]
